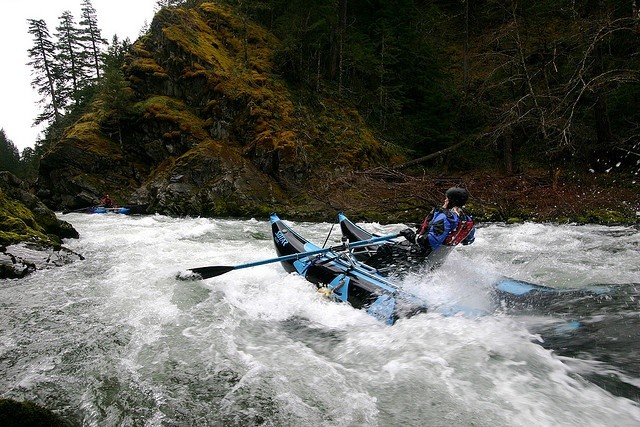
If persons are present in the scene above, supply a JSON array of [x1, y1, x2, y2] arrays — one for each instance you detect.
[[100, 195, 113, 208], [389, 186, 475, 267]]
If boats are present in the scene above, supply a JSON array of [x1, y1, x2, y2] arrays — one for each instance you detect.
[[269, 212, 640, 351], [88, 205, 131, 214]]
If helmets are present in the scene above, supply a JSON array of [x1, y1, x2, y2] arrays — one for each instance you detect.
[[446, 187, 468, 209]]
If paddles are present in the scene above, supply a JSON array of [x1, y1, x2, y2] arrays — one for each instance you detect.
[[171, 234, 402, 282], [62, 203, 103, 215]]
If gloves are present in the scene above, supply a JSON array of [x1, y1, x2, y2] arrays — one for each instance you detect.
[[400, 229, 416, 244]]
